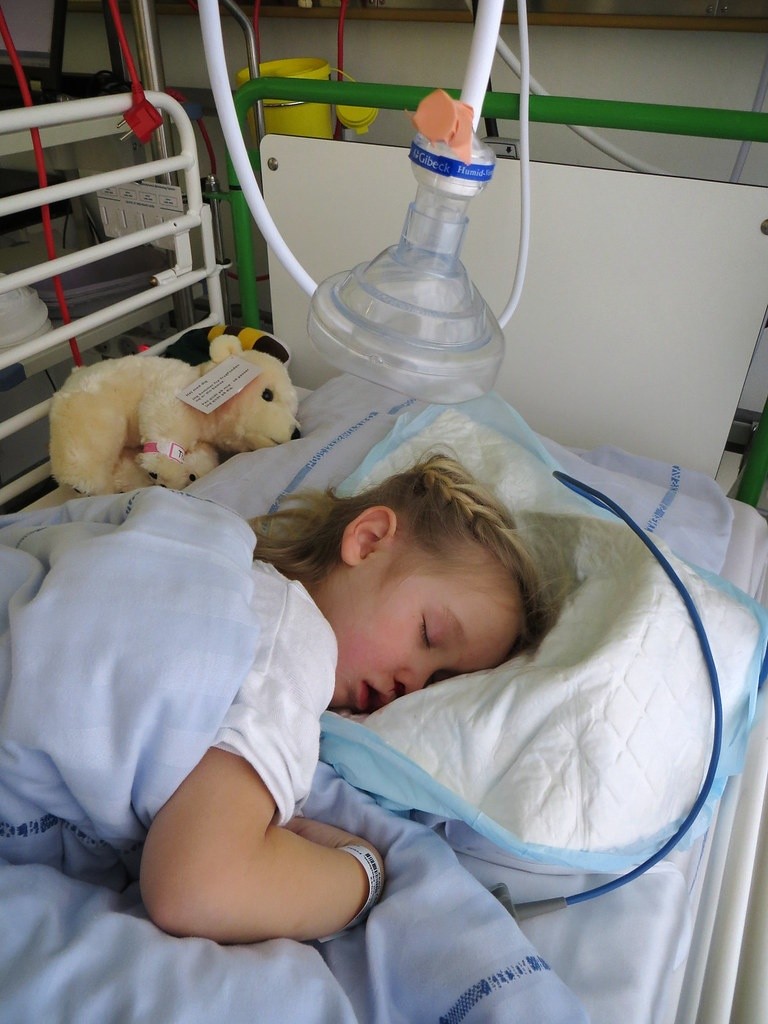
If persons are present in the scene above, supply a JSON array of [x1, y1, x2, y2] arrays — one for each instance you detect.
[[141, 453, 563, 943]]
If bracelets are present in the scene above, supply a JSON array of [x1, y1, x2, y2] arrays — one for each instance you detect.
[[317, 846, 382, 943]]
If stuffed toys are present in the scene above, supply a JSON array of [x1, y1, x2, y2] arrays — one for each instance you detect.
[[49, 321, 302, 494]]
